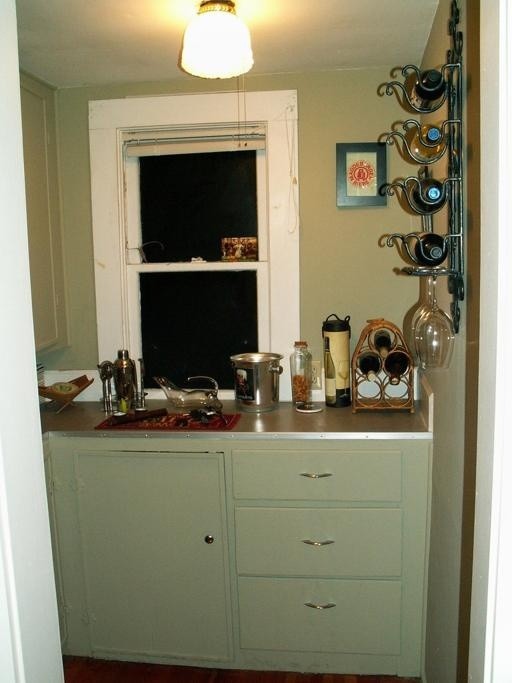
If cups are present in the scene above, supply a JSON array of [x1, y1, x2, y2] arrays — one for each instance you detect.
[[415, 267, 456, 373], [409, 267, 451, 367]]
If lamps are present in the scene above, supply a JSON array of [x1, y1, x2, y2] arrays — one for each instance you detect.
[[175, 0, 259, 86]]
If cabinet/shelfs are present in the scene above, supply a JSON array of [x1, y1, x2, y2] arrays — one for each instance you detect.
[[67, 423, 239, 678], [229, 426, 438, 678]]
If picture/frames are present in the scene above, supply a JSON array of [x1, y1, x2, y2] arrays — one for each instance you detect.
[[331, 140, 394, 214]]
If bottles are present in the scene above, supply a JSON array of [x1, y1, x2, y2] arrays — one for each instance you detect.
[[373, 329, 391, 359], [408, 233, 449, 267], [112, 351, 136, 413], [290, 342, 313, 405], [358, 354, 381, 382], [386, 346, 409, 385], [405, 124, 445, 165], [407, 68, 448, 112], [322, 315, 352, 408], [322, 335, 336, 404], [409, 178, 447, 214]]
